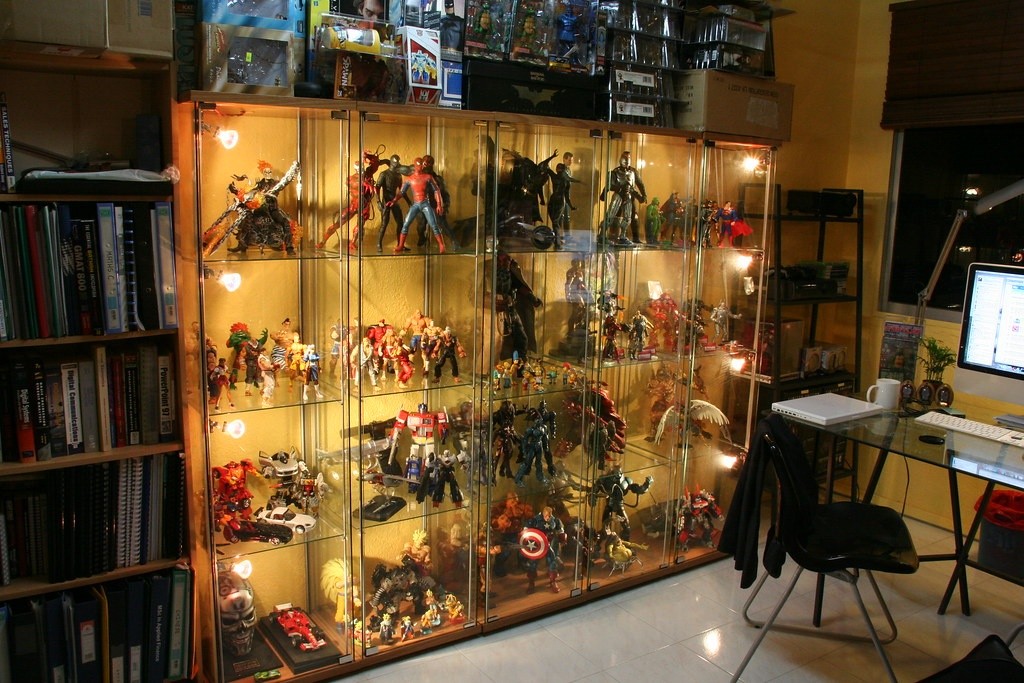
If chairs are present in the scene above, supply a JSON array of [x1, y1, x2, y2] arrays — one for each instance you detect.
[[918, 622, 1024, 683], [728, 411, 919, 683]]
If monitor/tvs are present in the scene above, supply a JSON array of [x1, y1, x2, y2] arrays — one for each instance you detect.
[[945, 449, 1024, 488], [956, 262, 1024, 381]]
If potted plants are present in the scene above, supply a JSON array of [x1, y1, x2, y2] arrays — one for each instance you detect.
[[908, 328, 957, 407]]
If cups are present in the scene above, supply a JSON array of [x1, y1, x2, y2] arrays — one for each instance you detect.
[[866, 378, 901, 411]]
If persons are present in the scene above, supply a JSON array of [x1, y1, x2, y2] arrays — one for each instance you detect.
[[320, 491, 567, 646], [185, 317, 324, 406], [602, 293, 743, 364], [226, 160, 296, 256], [547, 152, 582, 251], [318, 150, 449, 253], [328, 308, 467, 383], [388, 403, 463, 510], [647, 190, 753, 248], [595, 152, 648, 246], [480, 396, 557, 487], [354, 0, 384, 20], [566, 258, 593, 329]]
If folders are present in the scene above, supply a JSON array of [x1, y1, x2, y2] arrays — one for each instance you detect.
[[168, 568, 187, 683], [8, 597, 41, 683], [92, 201, 121, 335], [106, 581, 126, 683], [125, 579, 145, 683], [40, 591, 68, 683], [72, 585, 100, 683], [146, 574, 167, 683], [151, 202, 178, 329]]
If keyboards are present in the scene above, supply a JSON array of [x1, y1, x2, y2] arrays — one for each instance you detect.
[[914, 411, 1024, 448]]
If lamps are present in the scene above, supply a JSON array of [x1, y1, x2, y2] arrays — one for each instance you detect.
[[855, 173, 1024, 502]]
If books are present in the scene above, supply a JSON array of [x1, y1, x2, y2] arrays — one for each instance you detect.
[[0, 102, 197, 683]]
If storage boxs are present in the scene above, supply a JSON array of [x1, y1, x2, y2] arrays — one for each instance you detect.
[[394, 26, 443, 107], [0, 0, 794, 142], [782, 417, 846, 479], [739, 315, 848, 383], [750, 258, 850, 302]]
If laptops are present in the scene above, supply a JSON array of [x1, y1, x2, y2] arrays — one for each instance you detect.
[[772, 393, 884, 425]]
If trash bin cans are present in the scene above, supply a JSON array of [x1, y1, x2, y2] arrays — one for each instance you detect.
[[978, 490, 1024, 580]]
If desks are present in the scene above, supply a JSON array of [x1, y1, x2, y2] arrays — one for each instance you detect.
[[762, 388, 1024, 629]]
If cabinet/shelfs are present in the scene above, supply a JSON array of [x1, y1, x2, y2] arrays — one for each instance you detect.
[[712, 182, 864, 534], [0, 37, 220, 683], [186, 89, 781, 683]]
